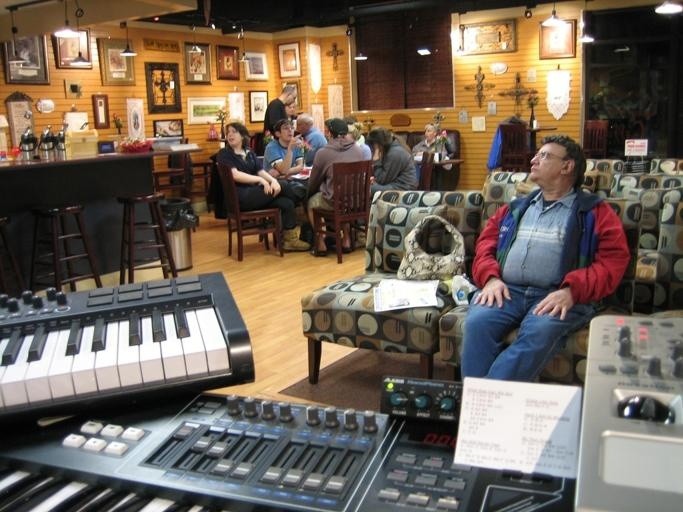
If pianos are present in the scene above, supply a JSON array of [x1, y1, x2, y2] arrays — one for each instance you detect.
[[1, 272, 255, 427]]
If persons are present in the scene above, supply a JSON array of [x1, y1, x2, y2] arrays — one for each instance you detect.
[[221, 111, 454, 255], [263, 84, 298, 142], [459, 134, 629, 384]]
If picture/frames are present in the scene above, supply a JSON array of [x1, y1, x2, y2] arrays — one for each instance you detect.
[[459, 17, 515, 55], [539, 19, 576, 60]]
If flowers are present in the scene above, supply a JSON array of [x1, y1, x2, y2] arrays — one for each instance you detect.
[[525, 89, 539, 112]]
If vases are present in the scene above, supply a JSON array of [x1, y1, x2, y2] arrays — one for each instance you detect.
[[530, 112, 535, 128]]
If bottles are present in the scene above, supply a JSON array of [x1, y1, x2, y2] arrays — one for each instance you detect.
[[20, 125, 66, 163], [207, 124, 219, 142]]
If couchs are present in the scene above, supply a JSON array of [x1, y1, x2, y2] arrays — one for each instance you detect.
[[361, 130, 460, 191]]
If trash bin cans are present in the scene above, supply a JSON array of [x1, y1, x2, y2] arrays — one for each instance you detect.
[[159, 197, 192, 270]]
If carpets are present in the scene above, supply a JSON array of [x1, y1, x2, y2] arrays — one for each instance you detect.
[[278, 347, 459, 415]]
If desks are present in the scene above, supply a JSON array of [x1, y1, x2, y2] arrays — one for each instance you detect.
[[525, 124, 557, 153]]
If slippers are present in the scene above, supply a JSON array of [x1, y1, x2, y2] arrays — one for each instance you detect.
[[342, 245, 352, 253], [310, 245, 328, 256]]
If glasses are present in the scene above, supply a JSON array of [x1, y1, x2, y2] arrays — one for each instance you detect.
[[534, 151, 566, 161]]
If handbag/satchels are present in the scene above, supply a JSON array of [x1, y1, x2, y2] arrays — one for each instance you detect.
[[396, 215, 467, 282]]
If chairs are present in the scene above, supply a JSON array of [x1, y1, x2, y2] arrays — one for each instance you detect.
[[583, 120, 610, 158], [153, 137, 214, 232], [499, 124, 539, 172], [216, 161, 284, 261]]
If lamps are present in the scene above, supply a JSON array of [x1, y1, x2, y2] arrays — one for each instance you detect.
[[542, 0, 564, 26], [654, 0, 683, 14]]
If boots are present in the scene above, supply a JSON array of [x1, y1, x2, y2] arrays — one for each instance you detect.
[[282, 223, 311, 252]]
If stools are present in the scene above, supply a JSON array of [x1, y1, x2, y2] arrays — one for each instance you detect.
[[116, 191, 178, 284], [0, 213, 26, 294], [29, 201, 102, 297]]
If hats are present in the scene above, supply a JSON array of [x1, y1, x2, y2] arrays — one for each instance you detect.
[[324, 117, 348, 139], [366, 126, 392, 146]]
